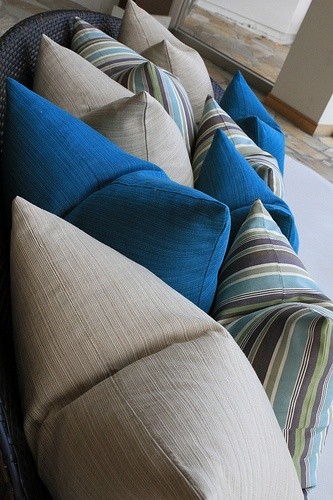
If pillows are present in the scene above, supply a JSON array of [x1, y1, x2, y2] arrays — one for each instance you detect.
[[194, 128, 300, 255], [218, 70, 286, 179], [8, 193, 307, 500], [2, 75, 230, 316], [33, 32, 194, 189], [70, 14, 194, 161], [117, 0, 214, 120], [192, 93, 286, 199], [211, 198, 333, 499]]
